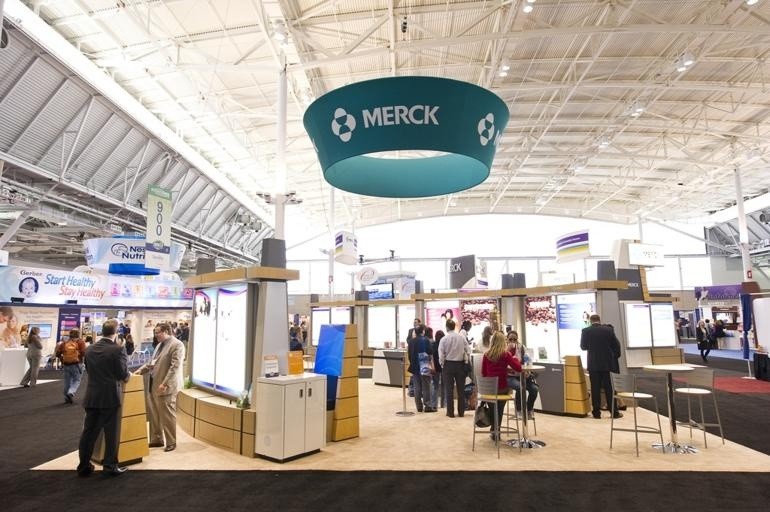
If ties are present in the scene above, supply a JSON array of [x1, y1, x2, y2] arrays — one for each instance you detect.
[[153, 342, 164, 359]]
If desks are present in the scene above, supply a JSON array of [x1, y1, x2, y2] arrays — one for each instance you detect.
[[505, 364, 547, 450], [642, 361, 706, 454]]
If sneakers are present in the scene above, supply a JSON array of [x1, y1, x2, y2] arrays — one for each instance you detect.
[[614, 404, 627, 418], [528, 410, 535, 420]]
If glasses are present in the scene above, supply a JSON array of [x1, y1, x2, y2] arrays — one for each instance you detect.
[[509, 338, 517, 341]]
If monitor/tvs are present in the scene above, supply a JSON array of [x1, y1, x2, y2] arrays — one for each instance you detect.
[[28, 323, 53, 339], [365, 283, 393, 301], [712, 311, 735, 326]]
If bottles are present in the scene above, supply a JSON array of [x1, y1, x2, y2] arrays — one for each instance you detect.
[[523, 352, 533, 368]]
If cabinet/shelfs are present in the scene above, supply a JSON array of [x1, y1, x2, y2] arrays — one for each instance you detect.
[[253, 372, 329, 466]]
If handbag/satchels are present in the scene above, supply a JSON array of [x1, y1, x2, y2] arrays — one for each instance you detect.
[[475, 401, 491, 428], [418, 352, 436, 377]]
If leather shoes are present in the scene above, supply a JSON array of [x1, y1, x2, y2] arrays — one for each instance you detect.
[[149, 442, 176, 451], [78, 465, 128, 476]]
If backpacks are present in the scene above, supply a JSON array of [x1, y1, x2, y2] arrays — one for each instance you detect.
[[62, 338, 81, 364]]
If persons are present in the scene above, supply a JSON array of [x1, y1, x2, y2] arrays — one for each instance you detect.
[[0, 307, 22, 348], [674, 318, 728, 362], [20, 320, 189, 477], [601, 324, 626, 410], [580, 315, 623, 418], [290, 321, 307, 355], [406, 319, 538, 441]]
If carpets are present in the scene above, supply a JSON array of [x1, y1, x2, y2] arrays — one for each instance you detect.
[[28, 377, 770, 476]]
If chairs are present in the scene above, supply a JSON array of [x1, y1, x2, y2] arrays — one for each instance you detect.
[[605, 371, 665, 458], [471, 351, 517, 436], [673, 367, 728, 451], [471, 376, 523, 460]]
